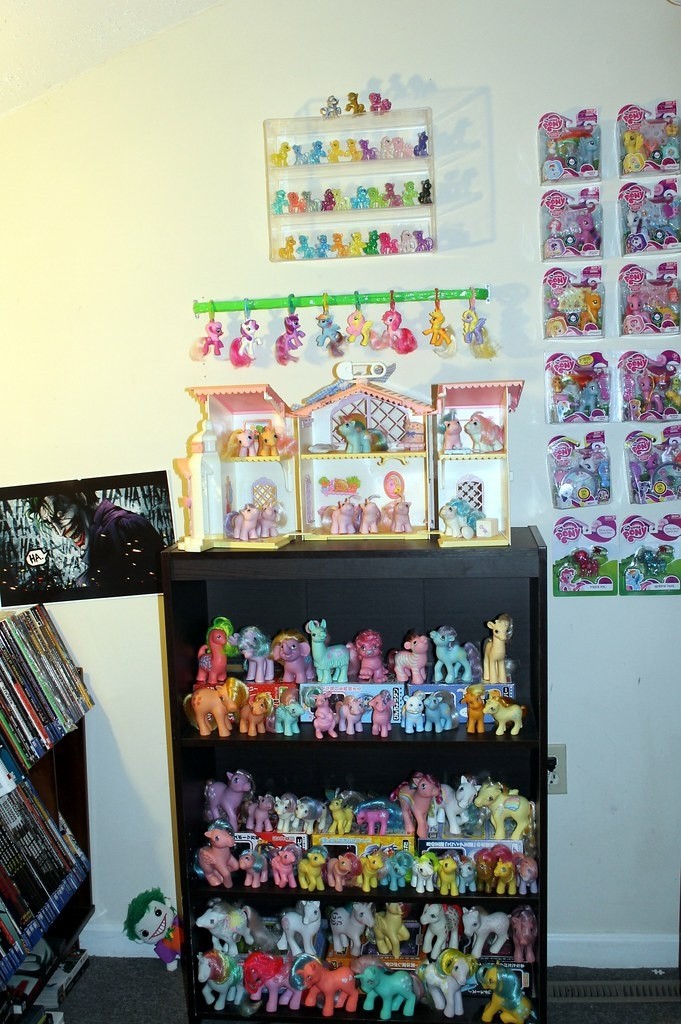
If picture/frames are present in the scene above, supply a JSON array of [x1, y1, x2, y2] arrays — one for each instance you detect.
[[0, 470, 176, 607]]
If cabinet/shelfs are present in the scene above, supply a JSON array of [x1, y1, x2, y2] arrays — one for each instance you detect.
[[0, 664, 96, 1024], [158, 523, 551, 1024]]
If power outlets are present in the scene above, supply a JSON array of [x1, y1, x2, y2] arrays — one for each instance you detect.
[[548, 744, 568, 795]]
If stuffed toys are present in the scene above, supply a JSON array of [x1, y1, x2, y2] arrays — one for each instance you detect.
[[123, 887, 184, 972]]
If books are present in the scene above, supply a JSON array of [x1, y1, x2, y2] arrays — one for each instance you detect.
[[0, 603, 95, 987]]
